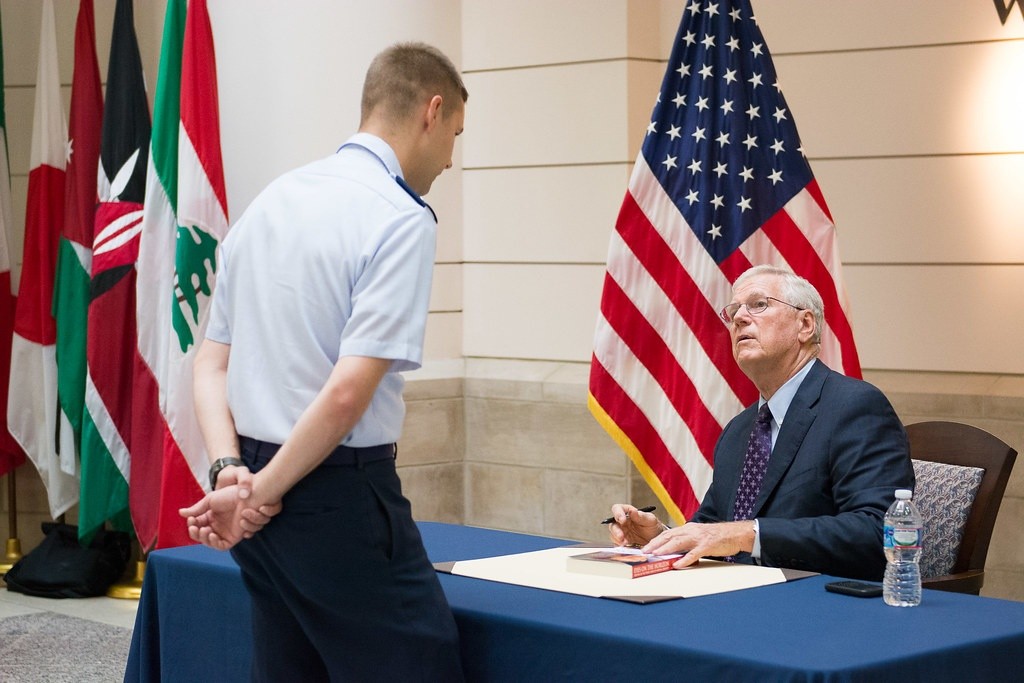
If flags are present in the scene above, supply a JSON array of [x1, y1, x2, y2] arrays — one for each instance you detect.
[[0, 0, 229, 553], [590, 1, 863, 526]]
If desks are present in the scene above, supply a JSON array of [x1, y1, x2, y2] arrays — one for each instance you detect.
[[123, 521, 1024, 683]]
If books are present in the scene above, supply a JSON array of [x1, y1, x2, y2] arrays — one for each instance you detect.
[[567, 546, 700, 581]]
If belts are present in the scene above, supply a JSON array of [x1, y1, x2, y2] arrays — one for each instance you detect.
[[238, 434, 397, 467]]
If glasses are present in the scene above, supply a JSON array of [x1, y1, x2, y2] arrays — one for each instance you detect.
[[719, 296, 806, 323]]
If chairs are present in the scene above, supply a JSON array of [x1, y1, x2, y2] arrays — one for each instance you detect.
[[903, 421, 1019, 596]]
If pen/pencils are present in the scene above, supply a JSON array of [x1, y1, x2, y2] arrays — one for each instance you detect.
[[601, 506, 656, 525]]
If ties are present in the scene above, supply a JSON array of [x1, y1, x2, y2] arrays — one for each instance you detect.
[[724, 403, 774, 564]]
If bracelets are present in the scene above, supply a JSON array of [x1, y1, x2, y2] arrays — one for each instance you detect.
[[209, 457, 246, 492]]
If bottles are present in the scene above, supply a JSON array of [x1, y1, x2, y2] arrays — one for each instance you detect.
[[883, 490, 922, 606]]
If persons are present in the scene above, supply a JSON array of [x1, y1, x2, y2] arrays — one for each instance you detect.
[[179, 40, 473, 682], [608, 264, 916, 582]]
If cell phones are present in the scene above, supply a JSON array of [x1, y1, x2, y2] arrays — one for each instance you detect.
[[824, 580, 883, 598]]
